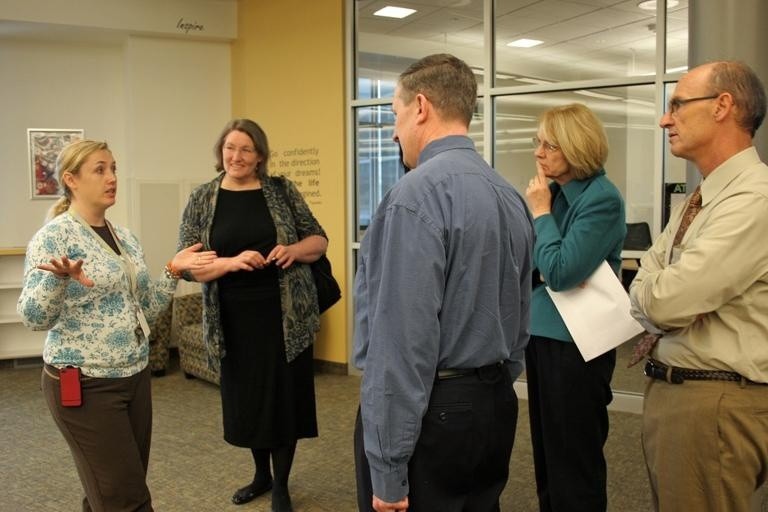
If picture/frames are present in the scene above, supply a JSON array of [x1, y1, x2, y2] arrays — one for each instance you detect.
[[27, 129, 85, 199]]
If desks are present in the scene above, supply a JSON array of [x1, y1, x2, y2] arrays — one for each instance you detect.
[[619, 250, 649, 269]]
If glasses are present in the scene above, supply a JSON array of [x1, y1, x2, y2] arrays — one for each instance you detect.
[[531, 137, 564, 154], [667, 94, 722, 115]]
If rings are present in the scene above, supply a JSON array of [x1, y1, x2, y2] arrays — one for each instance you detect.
[[199, 256, 200, 260]]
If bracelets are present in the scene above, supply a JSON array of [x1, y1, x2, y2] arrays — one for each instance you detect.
[[165, 261, 177, 274]]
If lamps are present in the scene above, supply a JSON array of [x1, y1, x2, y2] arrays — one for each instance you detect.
[[372, 5, 418, 20]]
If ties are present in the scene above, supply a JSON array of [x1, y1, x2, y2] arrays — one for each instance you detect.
[[626, 184, 706, 365]]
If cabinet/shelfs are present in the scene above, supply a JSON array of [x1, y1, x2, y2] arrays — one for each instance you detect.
[[0, 247, 56, 359]]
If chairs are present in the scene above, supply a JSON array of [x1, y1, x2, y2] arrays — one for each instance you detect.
[[623, 222, 652, 251]]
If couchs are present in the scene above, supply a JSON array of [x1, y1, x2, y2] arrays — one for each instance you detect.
[[138, 301, 173, 377], [174, 292, 224, 386]]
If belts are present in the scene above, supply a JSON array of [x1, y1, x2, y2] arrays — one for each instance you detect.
[[646, 357, 746, 384], [433, 363, 503, 381]]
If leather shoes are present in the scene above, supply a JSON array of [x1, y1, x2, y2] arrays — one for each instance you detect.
[[271, 483, 293, 512], [232, 475, 273, 504]]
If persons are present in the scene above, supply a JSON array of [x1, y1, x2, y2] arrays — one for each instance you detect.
[[350, 53, 537, 511], [16, 139, 218, 508], [629, 60, 768, 511], [177, 118, 329, 512], [525, 103, 627, 511]]
